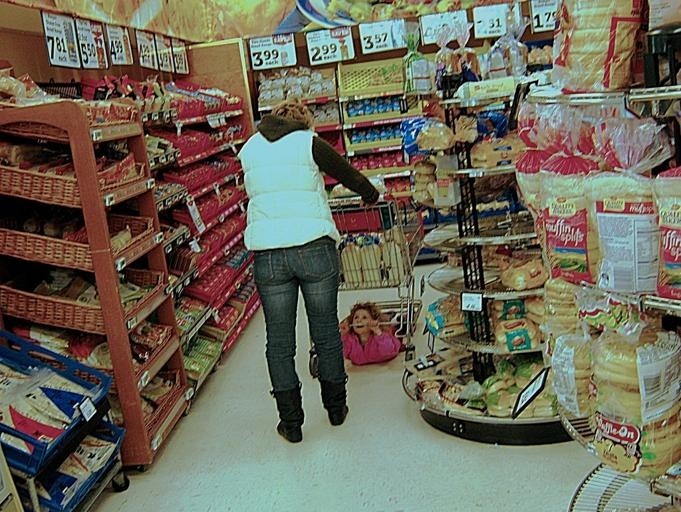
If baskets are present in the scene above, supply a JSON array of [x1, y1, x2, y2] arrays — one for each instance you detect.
[[0, 96, 139, 143], [33, 76, 85, 102], [0, 163, 146, 208], [0, 207, 155, 273], [0, 265, 166, 336], [4, 320, 176, 389], [334, 56, 408, 97], [108, 368, 182, 457], [13, 417, 128, 512], [0, 326, 116, 472]]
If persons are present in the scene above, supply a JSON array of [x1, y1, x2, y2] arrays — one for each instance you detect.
[[339, 302, 416, 365], [237, 97, 380, 443]]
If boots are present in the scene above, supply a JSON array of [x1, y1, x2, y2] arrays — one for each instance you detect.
[[318, 374, 349, 426], [270, 384, 304, 443]]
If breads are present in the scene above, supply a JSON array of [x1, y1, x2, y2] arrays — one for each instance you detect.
[[516, 147, 681, 500], [552, 0, 643, 91], [462, 214, 550, 290], [426, 295, 547, 350], [407, 347, 559, 417], [402, 36, 552, 98], [408, 115, 527, 218]]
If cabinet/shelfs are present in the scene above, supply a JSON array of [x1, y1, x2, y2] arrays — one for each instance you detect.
[[251, 62, 348, 204], [0, 66, 192, 470], [527, 79, 681, 512], [338, 54, 439, 266], [397, 85, 592, 445], [0, 318, 129, 512], [80, 80, 263, 393]]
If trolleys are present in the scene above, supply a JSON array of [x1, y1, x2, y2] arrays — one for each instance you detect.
[[305, 189, 425, 380]]
